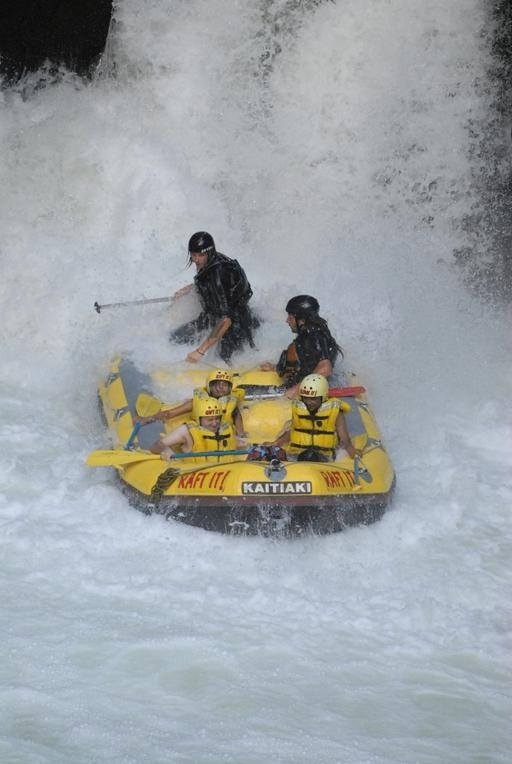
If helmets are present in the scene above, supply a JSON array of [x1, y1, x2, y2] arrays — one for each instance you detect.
[[286, 295, 320, 315], [205, 369, 234, 394], [299, 373, 330, 403], [188, 231, 215, 254], [195, 397, 223, 427]]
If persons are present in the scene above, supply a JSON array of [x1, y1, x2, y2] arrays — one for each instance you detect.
[[235, 294, 345, 401], [133, 367, 250, 437], [149, 396, 248, 464], [167, 231, 265, 367], [263, 373, 362, 462]]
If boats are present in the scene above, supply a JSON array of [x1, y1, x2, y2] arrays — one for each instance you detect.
[[97, 349, 395, 535]]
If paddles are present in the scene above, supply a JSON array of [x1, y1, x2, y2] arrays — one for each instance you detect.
[[244, 386, 366, 398], [124, 393, 162, 449], [350, 434, 367, 486], [87, 450, 247, 468]]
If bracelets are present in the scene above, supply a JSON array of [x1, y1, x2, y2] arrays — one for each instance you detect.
[[196, 348, 204, 355]]
